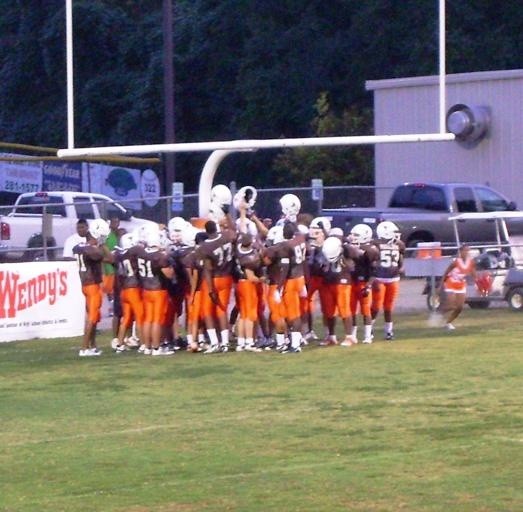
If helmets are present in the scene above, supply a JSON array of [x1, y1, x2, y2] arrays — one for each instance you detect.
[[119, 217, 193, 250], [208, 184, 232, 220], [321, 237, 344, 264], [308, 217, 331, 237], [233, 186, 257, 211], [235, 218, 309, 246], [279, 193, 301, 215], [328, 227, 344, 237], [376, 221, 401, 242], [347, 224, 372, 244], [87, 218, 110, 241]]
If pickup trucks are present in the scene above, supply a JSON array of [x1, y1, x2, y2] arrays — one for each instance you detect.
[[316, 181, 521, 259], [0, 190, 138, 258]]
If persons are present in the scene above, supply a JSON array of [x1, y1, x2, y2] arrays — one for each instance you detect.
[[62, 202, 408, 354], [432, 244, 478, 331]]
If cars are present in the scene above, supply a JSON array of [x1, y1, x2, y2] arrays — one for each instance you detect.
[[422, 247, 522, 311]]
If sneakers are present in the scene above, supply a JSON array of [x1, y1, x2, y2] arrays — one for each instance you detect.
[[77, 346, 102, 358], [110, 329, 318, 356], [317, 334, 338, 347], [385, 330, 394, 339], [444, 320, 455, 330], [340, 326, 374, 346]]
